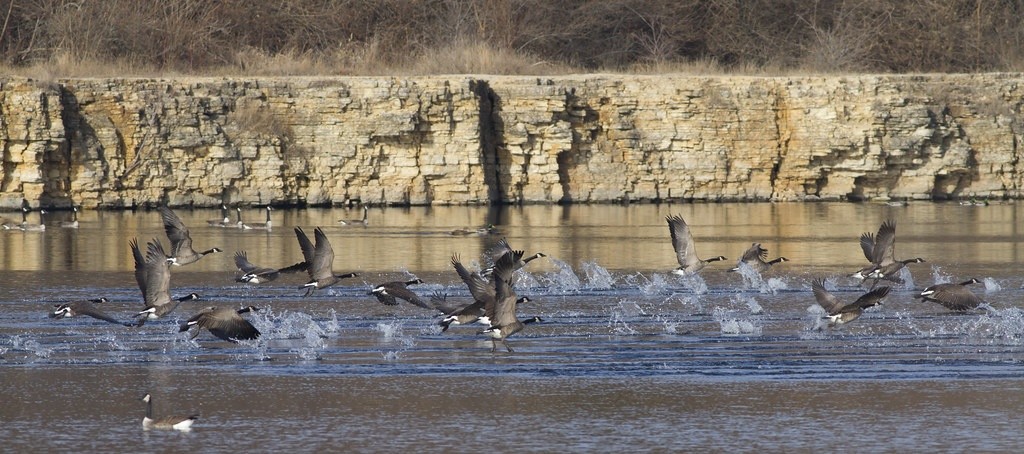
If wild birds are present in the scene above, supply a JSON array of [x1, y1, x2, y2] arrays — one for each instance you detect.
[[664, 213, 728, 277], [726, 242, 791, 274], [128, 236, 203, 328], [234, 251, 312, 284], [139, 393, 200, 431], [178, 305, 262, 343], [338, 206, 368, 227], [429, 237, 548, 353], [959, 198, 990, 206], [156, 205, 223, 267], [450, 223, 497, 237], [205, 202, 276, 230], [368, 279, 430, 309], [811, 278, 892, 327], [847, 219, 927, 292], [293, 226, 360, 298], [914, 278, 995, 311], [48, 298, 125, 326], [883, 200, 910, 206]]
